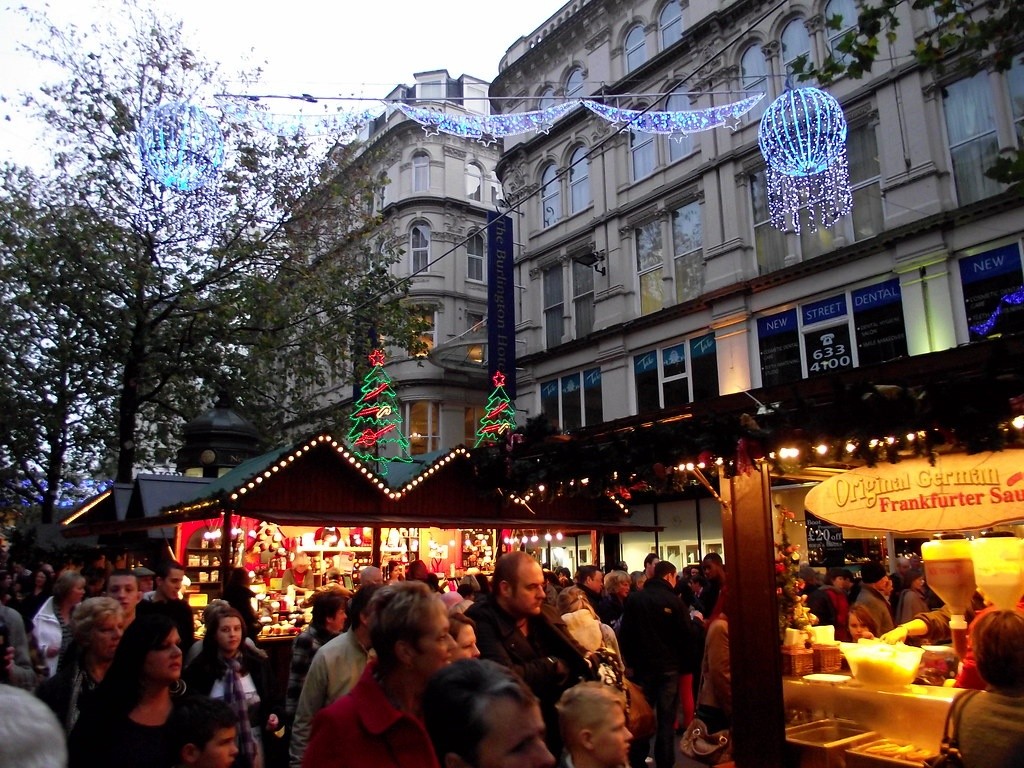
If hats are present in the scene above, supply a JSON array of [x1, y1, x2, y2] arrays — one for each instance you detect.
[[798, 565, 821, 583], [132, 567, 155, 576], [441, 592, 463, 610], [861, 562, 886, 584]]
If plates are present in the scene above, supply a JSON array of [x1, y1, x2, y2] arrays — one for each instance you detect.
[[804, 672, 851, 685]]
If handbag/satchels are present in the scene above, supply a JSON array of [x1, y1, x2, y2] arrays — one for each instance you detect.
[[623, 679, 655, 738], [923, 736, 964, 768], [679, 718, 732, 766]]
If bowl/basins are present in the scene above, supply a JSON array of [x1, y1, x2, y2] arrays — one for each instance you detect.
[[841, 641, 924, 691]]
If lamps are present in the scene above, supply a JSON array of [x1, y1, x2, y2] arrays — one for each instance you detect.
[[576, 251, 605, 276], [134, 86, 855, 236]]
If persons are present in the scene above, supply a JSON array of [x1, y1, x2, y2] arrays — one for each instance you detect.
[[797, 562, 990, 647], [283, 551, 736, 768], [952, 589, 1024, 768], [0, 546, 286, 768]]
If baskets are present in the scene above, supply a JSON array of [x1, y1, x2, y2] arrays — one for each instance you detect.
[[779, 645, 814, 677], [811, 641, 841, 674]]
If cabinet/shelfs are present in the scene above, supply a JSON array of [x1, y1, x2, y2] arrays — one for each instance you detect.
[[179, 529, 496, 596]]
